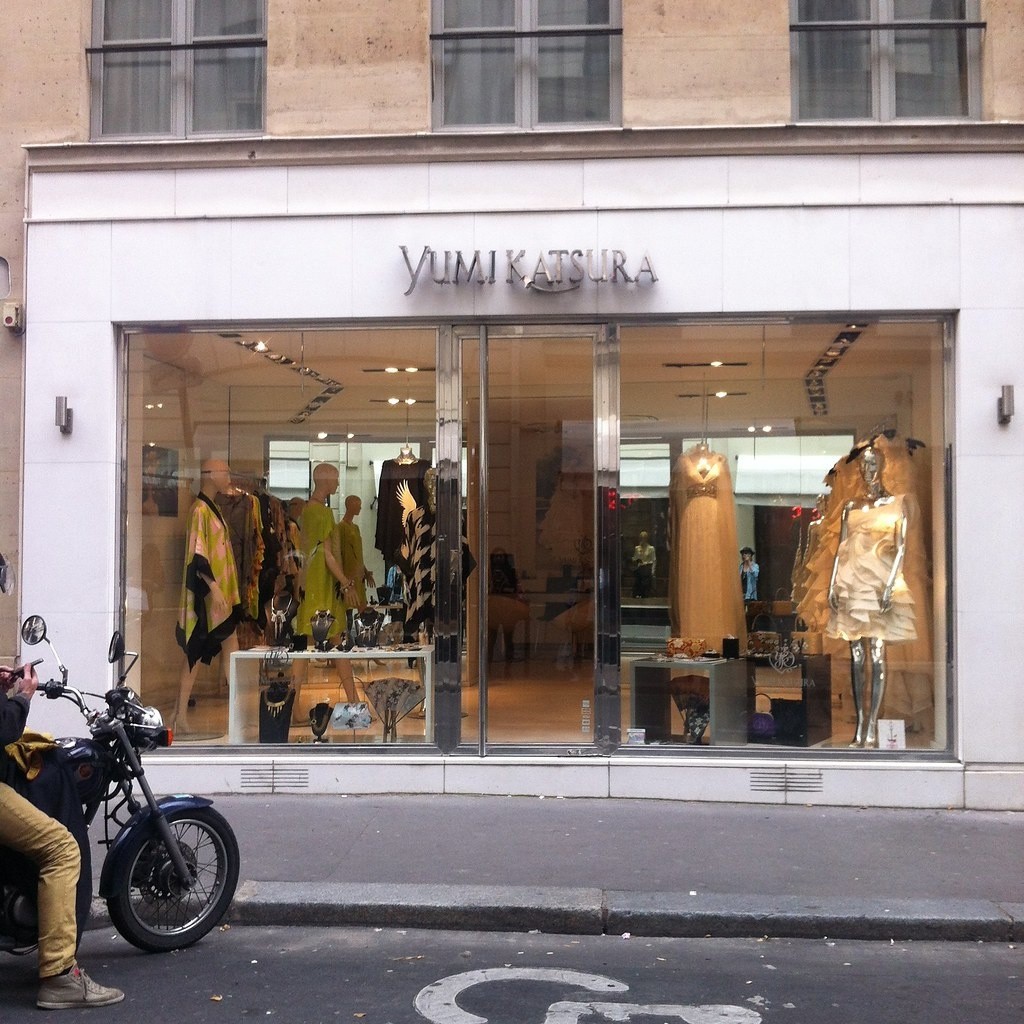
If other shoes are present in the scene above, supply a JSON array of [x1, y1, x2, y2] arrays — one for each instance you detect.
[[36, 962, 125, 1008], [291, 717, 310, 727], [406, 712, 425, 719], [461, 712, 468, 718]]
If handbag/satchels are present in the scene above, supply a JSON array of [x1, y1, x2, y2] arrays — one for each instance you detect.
[[330, 677, 372, 730], [666, 637, 706, 658], [790, 611, 823, 655], [769, 589, 793, 617], [746, 693, 777, 744], [684, 693, 711, 744], [747, 590, 771, 616], [746, 613, 779, 656], [488, 548, 517, 595], [769, 698, 806, 746]]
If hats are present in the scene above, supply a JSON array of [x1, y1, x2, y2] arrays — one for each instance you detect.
[[740, 547, 755, 556]]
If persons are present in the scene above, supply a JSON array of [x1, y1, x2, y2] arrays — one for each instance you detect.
[[828, 447, 918, 749], [669, 444, 748, 657], [284, 463, 377, 723], [739, 547, 759, 601], [0, 549, 125, 1008], [632, 532, 656, 598], [395, 468, 478, 716], [168, 457, 244, 735]]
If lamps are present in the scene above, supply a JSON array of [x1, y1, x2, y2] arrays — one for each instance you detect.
[[997, 386, 1015, 424], [55, 396, 73, 434]]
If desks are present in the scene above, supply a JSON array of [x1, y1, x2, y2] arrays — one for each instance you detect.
[[736, 653, 832, 748], [229, 645, 436, 744], [517, 591, 588, 662], [630, 653, 748, 747]]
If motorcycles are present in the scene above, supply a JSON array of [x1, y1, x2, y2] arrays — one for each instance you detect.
[[0, 616, 242, 976]]
